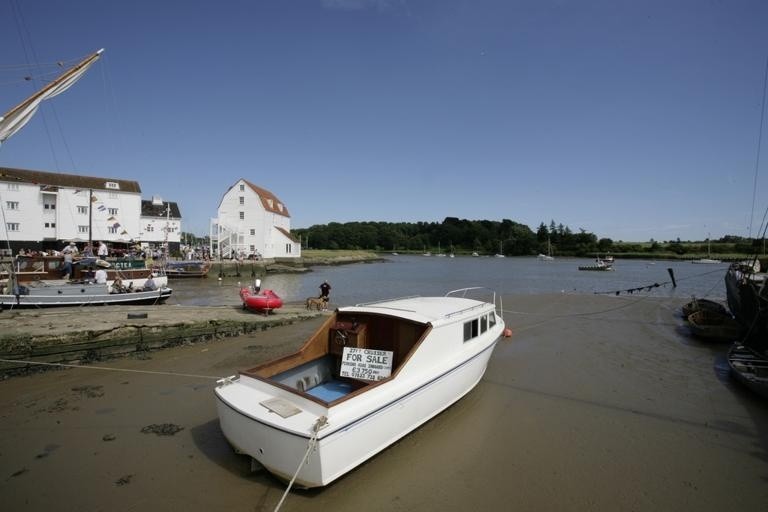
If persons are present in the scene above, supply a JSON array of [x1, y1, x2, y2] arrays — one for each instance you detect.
[[318, 279, 331, 311], [142, 273, 157, 292], [57, 240, 109, 282]]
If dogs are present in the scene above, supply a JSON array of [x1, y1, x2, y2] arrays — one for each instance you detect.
[[307, 295, 328, 311]]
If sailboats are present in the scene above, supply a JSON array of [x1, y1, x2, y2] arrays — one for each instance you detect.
[[424, 240, 455, 258], [0, 188, 208, 304]]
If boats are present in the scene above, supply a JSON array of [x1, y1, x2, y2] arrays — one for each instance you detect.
[[473, 252, 505, 258], [578, 255, 615, 271], [537, 254, 553, 261], [392, 253, 399, 256], [212, 286, 505, 489], [239, 286, 283, 313], [682, 258, 768, 395]]
